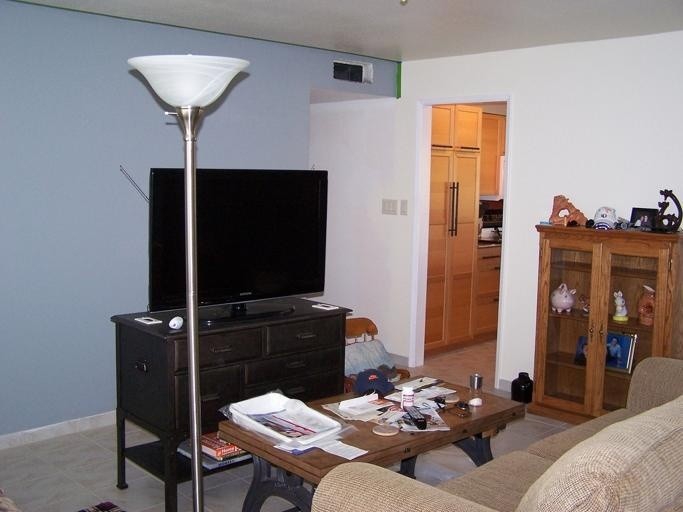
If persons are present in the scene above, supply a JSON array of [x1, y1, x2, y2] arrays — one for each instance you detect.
[[574, 344, 588, 366], [607, 336, 624, 367]]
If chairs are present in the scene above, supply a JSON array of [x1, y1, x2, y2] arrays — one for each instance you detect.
[[344, 318, 410, 392]]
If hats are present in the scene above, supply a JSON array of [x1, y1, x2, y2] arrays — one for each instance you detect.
[[592, 207, 617, 229], [355, 369, 394, 394]]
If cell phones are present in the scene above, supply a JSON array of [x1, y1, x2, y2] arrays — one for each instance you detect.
[[447, 407, 471, 418]]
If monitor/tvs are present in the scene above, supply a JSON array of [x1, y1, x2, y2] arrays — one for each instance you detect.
[[150, 167, 328, 323]]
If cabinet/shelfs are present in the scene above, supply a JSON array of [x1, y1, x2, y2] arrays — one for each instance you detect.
[[477, 245, 501, 343], [424, 104, 483, 359], [479, 113, 506, 196], [111, 299, 352, 512], [526, 224, 682, 426]]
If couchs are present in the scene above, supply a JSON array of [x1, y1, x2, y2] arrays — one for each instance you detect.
[[312, 357, 682, 512]]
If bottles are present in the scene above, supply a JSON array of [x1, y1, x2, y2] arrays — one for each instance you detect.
[[469, 372, 483, 410], [401, 386, 415, 409]]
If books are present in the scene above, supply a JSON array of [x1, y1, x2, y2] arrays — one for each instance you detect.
[[398, 407, 450, 433], [178, 432, 251, 471]]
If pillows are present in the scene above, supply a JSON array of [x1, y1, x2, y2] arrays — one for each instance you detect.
[[344, 340, 401, 382]]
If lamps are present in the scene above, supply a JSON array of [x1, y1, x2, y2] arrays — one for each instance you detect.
[[128, 54, 251, 512]]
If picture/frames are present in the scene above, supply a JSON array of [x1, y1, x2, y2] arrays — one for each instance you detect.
[[606, 332, 638, 374]]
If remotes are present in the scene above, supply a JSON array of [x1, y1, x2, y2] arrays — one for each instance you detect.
[[403, 405, 427, 430]]
